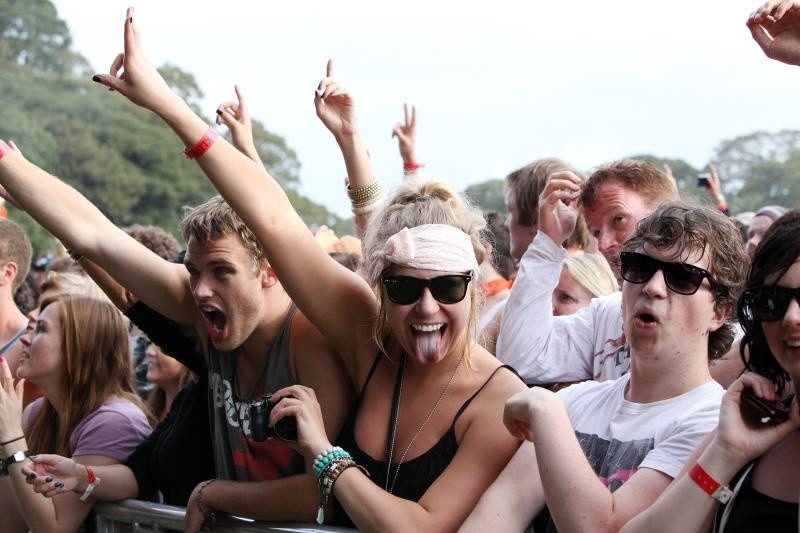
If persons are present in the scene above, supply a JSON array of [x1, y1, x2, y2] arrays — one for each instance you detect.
[[0, 6, 800, 533]]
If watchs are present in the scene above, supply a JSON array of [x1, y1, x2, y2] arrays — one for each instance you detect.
[[4, 450, 32, 466]]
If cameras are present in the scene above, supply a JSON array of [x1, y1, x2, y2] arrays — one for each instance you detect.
[[248, 393, 298, 441]]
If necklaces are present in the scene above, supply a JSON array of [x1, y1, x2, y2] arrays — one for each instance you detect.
[[385, 353, 466, 494]]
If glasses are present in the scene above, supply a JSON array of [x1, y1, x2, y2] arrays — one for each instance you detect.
[[750, 284, 800, 323], [381, 268, 475, 306], [620, 251, 725, 301]]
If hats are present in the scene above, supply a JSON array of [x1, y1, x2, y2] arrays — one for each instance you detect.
[[757, 206, 792, 223]]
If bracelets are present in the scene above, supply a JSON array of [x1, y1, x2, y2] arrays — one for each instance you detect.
[[403, 163, 424, 169], [352, 196, 380, 208], [67, 248, 84, 263], [183, 125, 219, 159], [710, 195, 725, 203], [0, 435, 26, 445], [687, 461, 733, 503], [312, 446, 343, 471], [315, 450, 352, 477], [73, 465, 101, 501], [719, 204, 727, 211], [346, 181, 379, 201], [196, 479, 219, 521], [352, 201, 380, 215], [316, 457, 371, 524]]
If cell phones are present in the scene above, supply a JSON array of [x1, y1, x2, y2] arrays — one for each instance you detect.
[[742, 392, 790, 422]]
